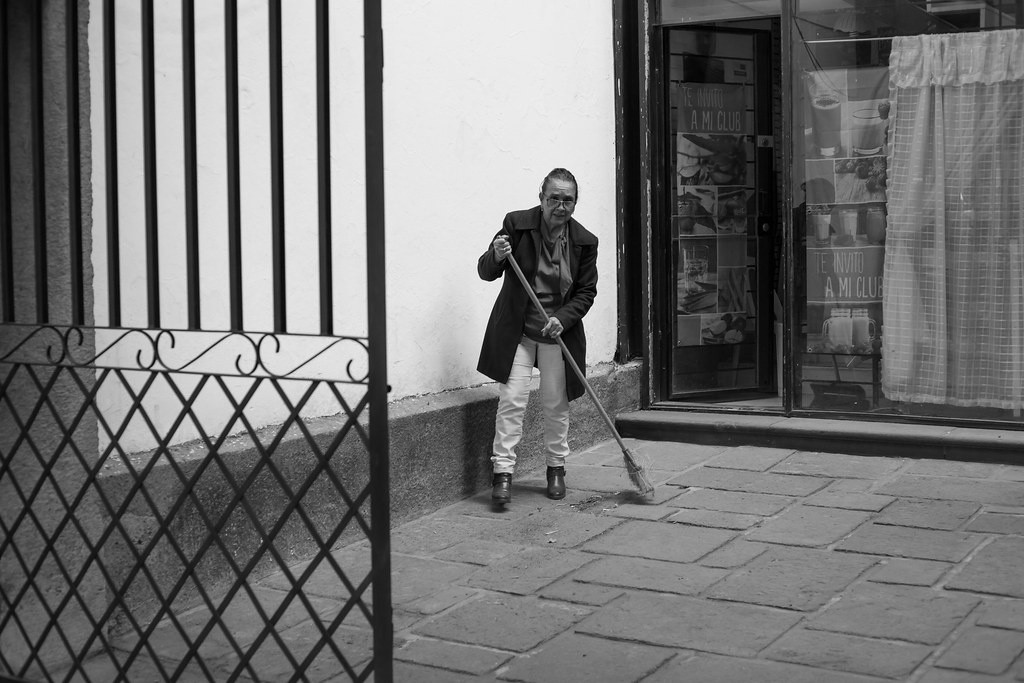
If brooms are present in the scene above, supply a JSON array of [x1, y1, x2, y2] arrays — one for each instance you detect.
[[497, 235, 656, 499]]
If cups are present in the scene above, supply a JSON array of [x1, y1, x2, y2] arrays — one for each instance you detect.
[[808, 80, 841, 156], [851, 108, 889, 154], [733, 207, 746, 232], [812, 214, 831, 245], [839, 209, 857, 245], [851, 308, 876, 346], [821, 308, 852, 353], [683, 245, 709, 296], [867, 207, 885, 243], [676, 199, 696, 234]]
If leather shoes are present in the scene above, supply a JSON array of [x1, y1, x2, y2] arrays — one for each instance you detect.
[[492, 473, 512, 503], [547, 466, 566, 499]]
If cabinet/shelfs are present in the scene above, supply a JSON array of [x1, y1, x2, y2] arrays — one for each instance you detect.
[[800, 71, 906, 412]]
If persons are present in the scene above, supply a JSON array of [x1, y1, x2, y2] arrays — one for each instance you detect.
[[476, 167, 599, 504]]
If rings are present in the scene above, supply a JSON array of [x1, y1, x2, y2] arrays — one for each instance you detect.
[[503, 248, 505, 252]]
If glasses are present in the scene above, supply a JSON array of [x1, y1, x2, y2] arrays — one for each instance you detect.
[[543, 192, 577, 210]]
